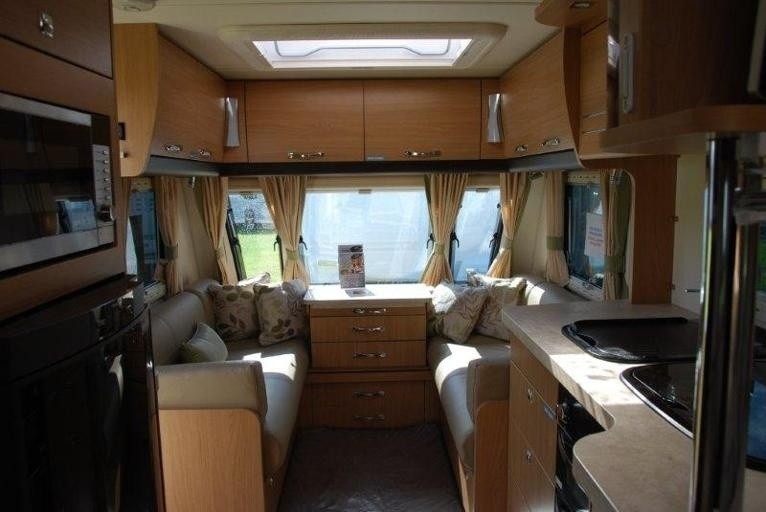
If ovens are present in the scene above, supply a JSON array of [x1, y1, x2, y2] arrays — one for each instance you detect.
[[549, 382, 608, 510]]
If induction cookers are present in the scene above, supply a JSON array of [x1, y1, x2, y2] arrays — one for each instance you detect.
[[628, 356, 766, 472]]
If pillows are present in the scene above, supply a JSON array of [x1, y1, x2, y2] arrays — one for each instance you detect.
[[207, 273, 270, 343], [426, 279, 488, 341], [180, 320, 229, 362], [254, 279, 311, 348], [467, 269, 526, 342]]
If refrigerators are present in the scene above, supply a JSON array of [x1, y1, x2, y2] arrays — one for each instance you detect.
[[2, 272, 169, 510]]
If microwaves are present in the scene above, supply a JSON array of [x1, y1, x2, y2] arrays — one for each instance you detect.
[[0, 92, 118, 280]]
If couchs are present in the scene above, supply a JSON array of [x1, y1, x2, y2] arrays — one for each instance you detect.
[[149, 278, 310, 512], [427, 272, 590, 512]]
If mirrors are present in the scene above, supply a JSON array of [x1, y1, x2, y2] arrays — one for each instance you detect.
[[126, 176, 169, 304]]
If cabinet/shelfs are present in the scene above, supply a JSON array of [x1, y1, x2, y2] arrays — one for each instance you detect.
[[507, 332, 559, 512], [297, 283, 441, 429], [111, 0, 766, 305]]
